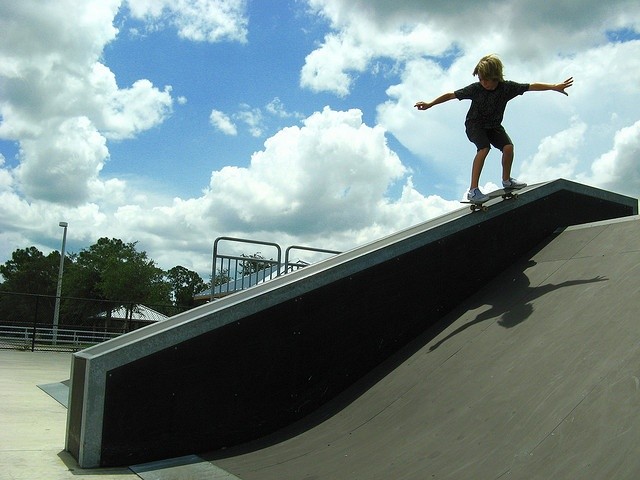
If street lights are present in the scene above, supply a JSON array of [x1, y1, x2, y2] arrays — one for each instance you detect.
[[53, 222, 67, 345]]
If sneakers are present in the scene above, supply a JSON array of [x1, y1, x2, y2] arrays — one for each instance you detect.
[[502, 179, 527, 189], [468, 189, 490, 202]]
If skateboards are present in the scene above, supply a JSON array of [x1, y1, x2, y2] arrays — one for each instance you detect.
[[460, 186, 526, 212]]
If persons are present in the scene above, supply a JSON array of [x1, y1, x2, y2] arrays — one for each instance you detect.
[[413, 56, 573, 202]]
